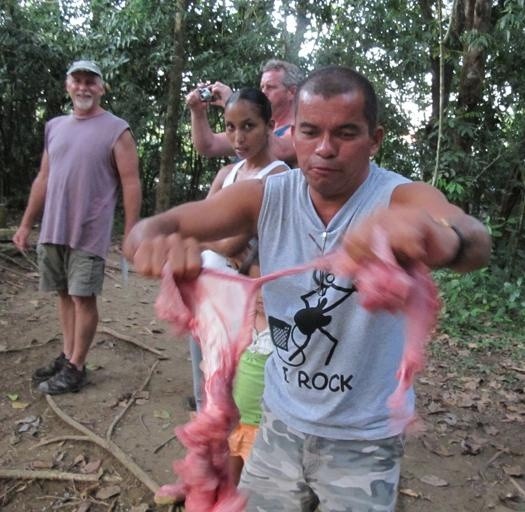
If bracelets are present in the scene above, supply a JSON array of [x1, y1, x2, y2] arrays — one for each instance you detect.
[[432, 215, 463, 269]]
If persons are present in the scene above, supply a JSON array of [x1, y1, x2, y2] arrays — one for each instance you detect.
[[184, 58, 302, 168], [120, 66, 492, 511], [12, 59, 143, 397], [185, 85, 289, 418], [219, 250, 279, 494]]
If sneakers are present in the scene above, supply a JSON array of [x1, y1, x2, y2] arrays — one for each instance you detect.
[[38, 361, 88, 394], [155, 483, 186, 506], [32, 352, 69, 380]]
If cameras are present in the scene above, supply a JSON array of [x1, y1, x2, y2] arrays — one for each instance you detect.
[[199, 87, 216, 102]]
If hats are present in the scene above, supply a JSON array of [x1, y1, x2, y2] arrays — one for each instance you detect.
[[67, 60, 103, 80]]
